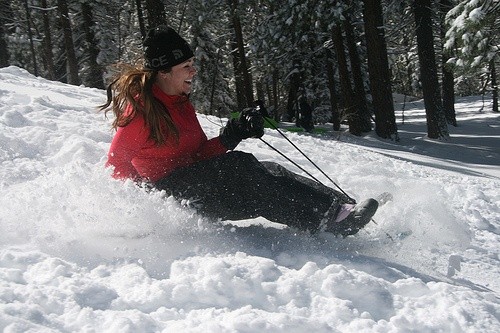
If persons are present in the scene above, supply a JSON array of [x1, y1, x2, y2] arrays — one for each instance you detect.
[[105, 25, 379, 239]]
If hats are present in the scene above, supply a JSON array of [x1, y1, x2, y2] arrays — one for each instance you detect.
[[142, 25, 195, 71]]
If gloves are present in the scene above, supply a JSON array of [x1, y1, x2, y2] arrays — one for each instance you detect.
[[219, 107, 262, 150]]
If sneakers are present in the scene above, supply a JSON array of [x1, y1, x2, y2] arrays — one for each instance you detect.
[[326, 198, 378, 238]]
